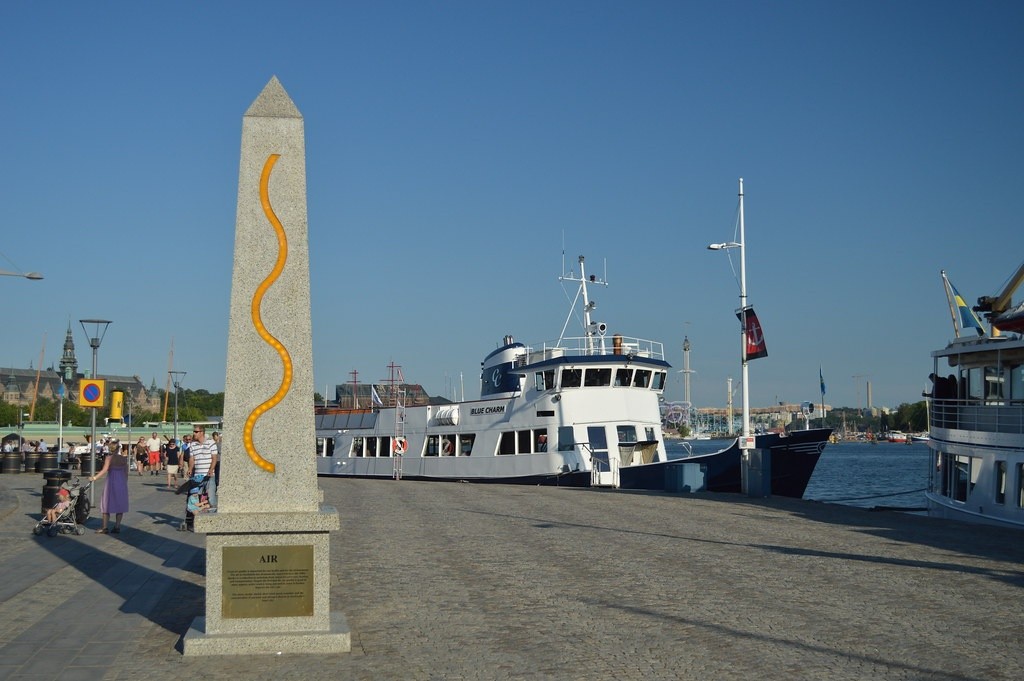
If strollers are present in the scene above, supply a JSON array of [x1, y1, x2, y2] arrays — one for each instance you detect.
[[33, 475, 94, 538]]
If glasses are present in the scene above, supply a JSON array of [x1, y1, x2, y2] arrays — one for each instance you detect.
[[169, 444, 174, 445], [194, 430, 203, 433], [186, 438, 191, 441]]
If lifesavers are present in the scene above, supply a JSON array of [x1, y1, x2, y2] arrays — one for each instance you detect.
[[392, 437, 409, 454]]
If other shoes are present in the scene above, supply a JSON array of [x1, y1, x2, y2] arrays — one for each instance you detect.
[[111, 525, 120, 534], [96, 527, 109, 533]]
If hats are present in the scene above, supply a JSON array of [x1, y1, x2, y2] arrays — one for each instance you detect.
[[189, 486, 199, 493]]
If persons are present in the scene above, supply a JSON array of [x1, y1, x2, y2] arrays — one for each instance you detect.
[[68, 446, 80, 462], [100, 437, 122, 454], [135, 437, 148, 476], [146, 432, 161, 476], [188, 425, 218, 505], [164, 434, 192, 488], [6, 440, 14, 452], [89, 441, 129, 534], [47, 489, 70, 526], [211, 432, 222, 486], [187, 486, 210, 515], [922, 373, 966, 428], [23, 439, 47, 452]]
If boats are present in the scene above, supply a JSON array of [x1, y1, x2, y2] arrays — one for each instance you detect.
[[927, 259, 1024, 531], [888, 430, 908, 442], [911, 435, 930, 442], [314, 227, 835, 501]]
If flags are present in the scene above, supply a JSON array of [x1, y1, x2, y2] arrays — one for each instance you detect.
[[737, 308, 768, 361], [947, 279, 985, 336], [373, 388, 383, 405], [820, 372, 825, 395]]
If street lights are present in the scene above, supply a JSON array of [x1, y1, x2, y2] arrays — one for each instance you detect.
[[78, 318, 113, 508], [167, 370, 187, 442], [706, 242, 750, 496]]
